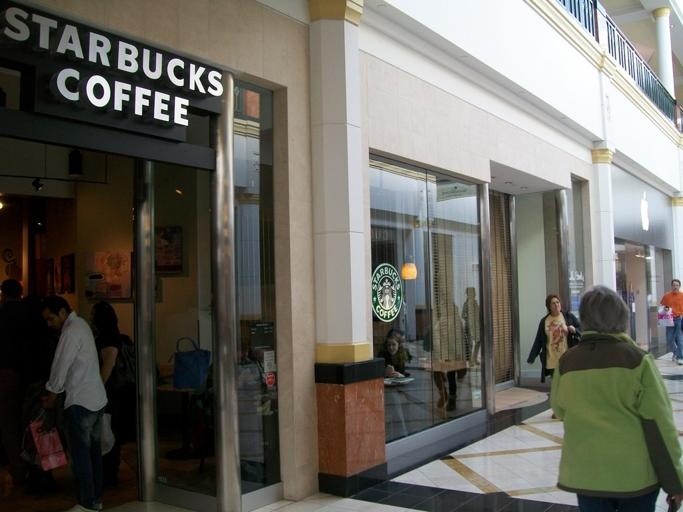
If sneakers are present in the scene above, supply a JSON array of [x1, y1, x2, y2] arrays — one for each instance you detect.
[[672, 353, 678, 361], [676, 355, 683, 365]]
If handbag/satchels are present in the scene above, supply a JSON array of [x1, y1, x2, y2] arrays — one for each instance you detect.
[[656, 308, 674, 328], [99, 410, 115, 458], [170, 337, 211, 389]]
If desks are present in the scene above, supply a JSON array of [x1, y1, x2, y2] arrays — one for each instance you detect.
[[383, 378, 416, 442], [406, 361, 469, 421]]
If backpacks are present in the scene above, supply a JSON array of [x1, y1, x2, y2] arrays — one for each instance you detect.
[[111, 333, 159, 389]]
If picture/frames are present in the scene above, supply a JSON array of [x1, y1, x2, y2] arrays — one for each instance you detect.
[[155, 223, 189, 277]]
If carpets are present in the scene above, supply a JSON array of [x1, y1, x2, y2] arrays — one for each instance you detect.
[[473, 387, 548, 414]]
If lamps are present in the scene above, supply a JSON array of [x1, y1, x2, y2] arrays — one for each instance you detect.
[[31, 179, 43, 192], [401, 256, 418, 279]]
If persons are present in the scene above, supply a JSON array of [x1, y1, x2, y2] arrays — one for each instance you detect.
[[549, 284, 682, 511], [38, 296, 108, 512], [0, 278, 136, 461], [422, 290, 471, 411], [461, 287, 483, 367], [525, 294, 580, 420], [659, 279, 682, 365], [377, 337, 411, 377]]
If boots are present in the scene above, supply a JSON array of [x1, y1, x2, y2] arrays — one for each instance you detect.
[[445, 395, 456, 411], [437, 390, 447, 408], [472, 351, 480, 366], [468, 352, 474, 367]]
